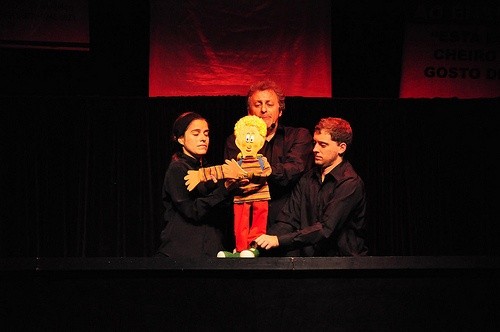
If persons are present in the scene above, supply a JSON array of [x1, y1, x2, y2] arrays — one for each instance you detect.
[[254, 117, 373, 257], [183, 117, 273, 257], [158, 111, 252, 258], [224, 83, 314, 257]]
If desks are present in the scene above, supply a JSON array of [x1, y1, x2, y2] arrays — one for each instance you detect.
[[0, 253, 500, 332]]
